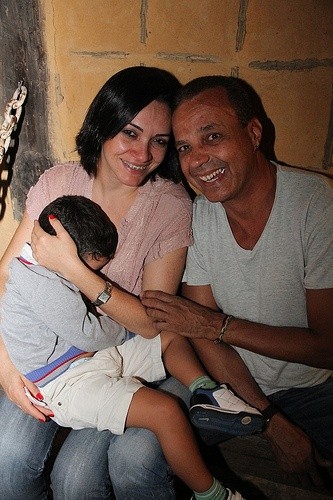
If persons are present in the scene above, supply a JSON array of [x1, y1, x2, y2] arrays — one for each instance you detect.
[[0, 66, 194, 500], [106, 75, 332, 500], [0, 195, 264, 500]]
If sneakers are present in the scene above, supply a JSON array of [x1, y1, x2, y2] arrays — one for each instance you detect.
[[189, 487, 245, 500], [189, 384, 266, 437]]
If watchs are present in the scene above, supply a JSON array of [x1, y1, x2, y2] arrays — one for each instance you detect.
[[90, 281, 113, 307]]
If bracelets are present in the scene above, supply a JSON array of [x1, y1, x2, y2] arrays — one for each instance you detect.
[[213, 315, 235, 345], [262, 404, 281, 427]]
